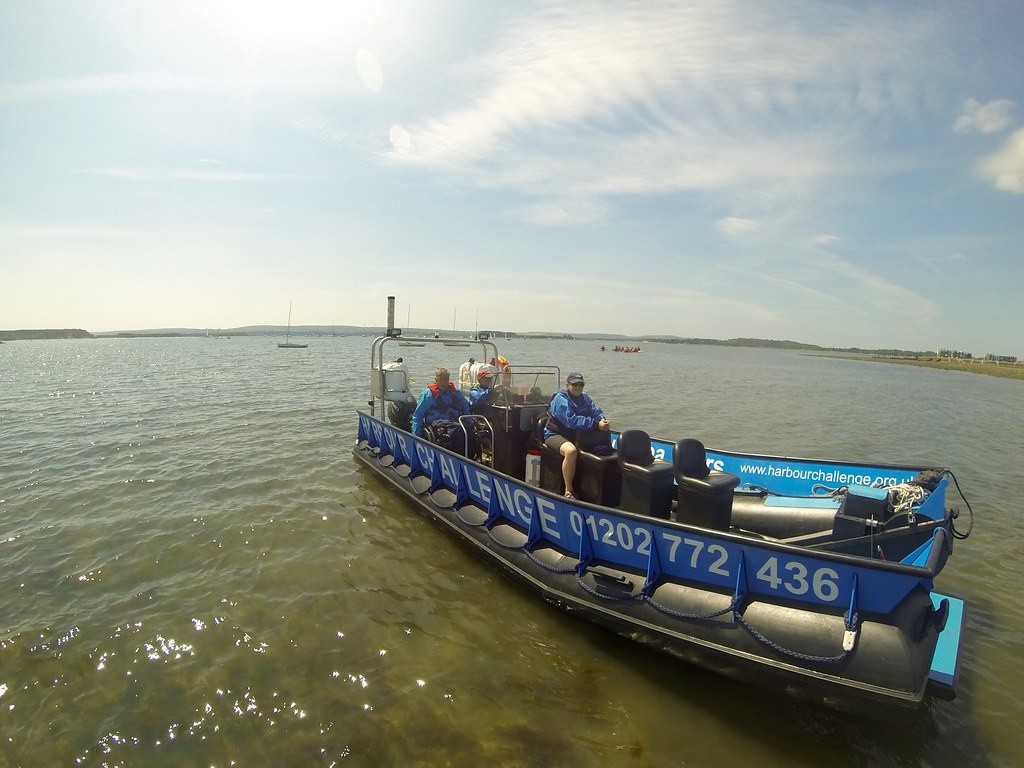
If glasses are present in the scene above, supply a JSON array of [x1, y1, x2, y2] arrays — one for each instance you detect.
[[569, 383, 584, 387]]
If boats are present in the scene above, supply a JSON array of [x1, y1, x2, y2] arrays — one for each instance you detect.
[[348, 296, 974, 720]]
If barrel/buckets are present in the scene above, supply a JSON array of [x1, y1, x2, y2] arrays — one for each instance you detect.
[[525, 450, 542, 488]]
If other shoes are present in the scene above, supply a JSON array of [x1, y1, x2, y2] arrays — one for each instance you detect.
[[564, 490, 575, 500]]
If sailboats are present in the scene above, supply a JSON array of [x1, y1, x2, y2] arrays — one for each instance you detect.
[[276, 300, 308, 347]]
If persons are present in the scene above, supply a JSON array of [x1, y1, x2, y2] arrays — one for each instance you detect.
[[615, 346, 640, 352], [412, 367, 473, 448], [544, 372, 610, 498], [470, 369, 501, 410]]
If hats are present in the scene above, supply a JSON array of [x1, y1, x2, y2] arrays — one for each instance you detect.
[[566, 372, 585, 384], [477, 369, 493, 381]]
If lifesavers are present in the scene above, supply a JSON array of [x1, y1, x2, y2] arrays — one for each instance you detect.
[[489, 355, 512, 387]]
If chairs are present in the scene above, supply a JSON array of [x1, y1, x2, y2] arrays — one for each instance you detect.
[[672, 438, 741, 533], [535, 418, 566, 496], [616, 429, 674, 520], [574, 416, 620, 508]]
